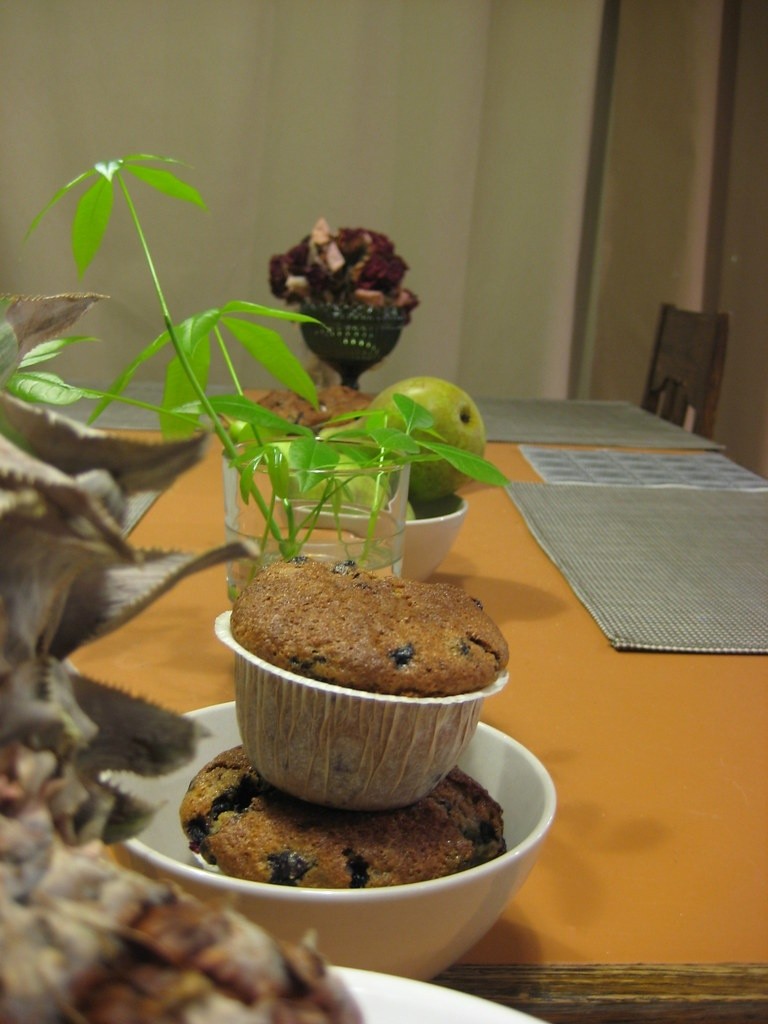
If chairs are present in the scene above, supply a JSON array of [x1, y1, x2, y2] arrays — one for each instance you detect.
[[639, 302, 731, 438]]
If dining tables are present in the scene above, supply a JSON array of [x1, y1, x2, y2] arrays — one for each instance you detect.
[[68, 397, 768, 1023]]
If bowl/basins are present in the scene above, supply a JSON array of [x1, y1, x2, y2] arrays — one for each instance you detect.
[[94, 696, 557, 985], [274, 495, 471, 592]]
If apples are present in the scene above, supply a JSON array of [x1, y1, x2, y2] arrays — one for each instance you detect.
[[293, 377, 484, 521]]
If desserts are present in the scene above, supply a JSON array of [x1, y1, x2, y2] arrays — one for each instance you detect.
[[210, 555, 509, 811], [255, 386, 375, 437], [179, 743, 506, 888]]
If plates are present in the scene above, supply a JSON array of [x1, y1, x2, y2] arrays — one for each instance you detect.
[[322, 966, 548, 1024]]
